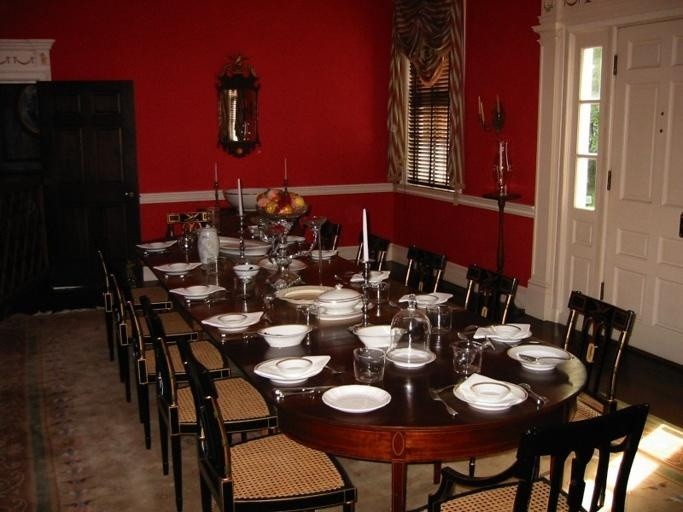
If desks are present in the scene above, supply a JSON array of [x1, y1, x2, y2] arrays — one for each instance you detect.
[[130, 230, 588, 511]]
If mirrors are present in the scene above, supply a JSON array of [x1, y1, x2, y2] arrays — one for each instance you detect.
[[217, 53, 260, 159]]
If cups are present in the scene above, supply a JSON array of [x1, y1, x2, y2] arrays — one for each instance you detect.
[[425, 304, 450, 335], [448, 341, 483, 376], [351, 347, 385, 384]]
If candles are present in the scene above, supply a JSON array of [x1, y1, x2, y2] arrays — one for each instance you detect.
[[478, 95, 482, 113], [283, 158, 289, 179], [496, 94, 500, 116], [236, 177, 245, 215], [358, 205, 371, 262], [213, 162, 219, 181], [480, 101, 486, 119]]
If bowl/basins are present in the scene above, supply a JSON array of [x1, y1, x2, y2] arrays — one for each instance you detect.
[[257, 323, 314, 348], [356, 325, 404, 350], [223, 187, 270, 211]]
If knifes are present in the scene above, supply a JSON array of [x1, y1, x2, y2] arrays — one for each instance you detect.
[[272, 385, 336, 398]]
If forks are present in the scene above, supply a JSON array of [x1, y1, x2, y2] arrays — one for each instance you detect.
[[426, 385, 458, 416]]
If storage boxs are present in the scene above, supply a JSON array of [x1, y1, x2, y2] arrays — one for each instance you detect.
[[0, 37, 58, 85]]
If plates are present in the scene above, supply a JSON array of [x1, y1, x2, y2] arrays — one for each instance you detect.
[[200, 311, 265, 332], [505, 346, 571, 372], [452, 372, 530, 413], [135, 234, 392, 321], [472, 322, 533, 344], [320, 384, 393, 414], [398, 291, 454, 310], [253, 351, 333, 387], [315, 313, 364, 322], [385, 348, 436, 369]]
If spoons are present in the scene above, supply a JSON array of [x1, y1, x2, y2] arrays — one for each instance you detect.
[[516, 353, 574, 362], [518, 380, 544, 404], [324, 366, 344, 376]]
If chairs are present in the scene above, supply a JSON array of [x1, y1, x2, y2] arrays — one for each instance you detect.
[[139, 294, 280, 476], [463, 265, 519, 325], [355, 231, 391, 271], [560, 290, 635, 422], [177, 336, 357, 512], [97, 249, 170, 362], [316, 221, 340, 251], [102, 258, 200, 404], [402, 244, 447, 294], [428, 403, 648, 512], [117, 272, 232, 451]]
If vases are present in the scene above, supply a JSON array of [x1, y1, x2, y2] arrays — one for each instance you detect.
[[488, 139, 514, 199]]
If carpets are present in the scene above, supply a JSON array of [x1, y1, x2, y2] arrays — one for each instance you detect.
[[1, 306, 683, 512]]
